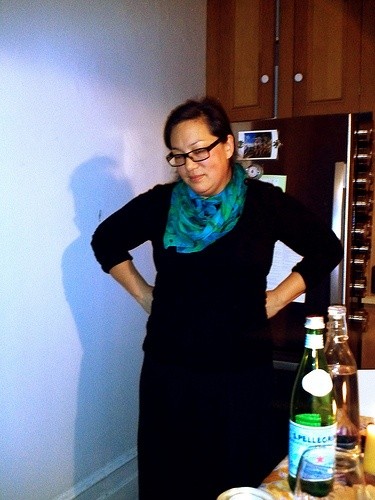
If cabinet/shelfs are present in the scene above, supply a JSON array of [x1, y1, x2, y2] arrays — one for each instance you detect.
[[206, 0, 375, 123]]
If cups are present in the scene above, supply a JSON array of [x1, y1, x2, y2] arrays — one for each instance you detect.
[[362, 424, 375, 478], [293, 445, 366, 499]]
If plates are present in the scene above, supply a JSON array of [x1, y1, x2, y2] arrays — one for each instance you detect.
[[216, 486, 273, 500]]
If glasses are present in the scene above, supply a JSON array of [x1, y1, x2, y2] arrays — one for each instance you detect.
[[165, 136, 223, 167]]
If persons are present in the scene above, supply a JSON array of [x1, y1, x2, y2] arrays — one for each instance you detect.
[[91, 98, 343, 500]]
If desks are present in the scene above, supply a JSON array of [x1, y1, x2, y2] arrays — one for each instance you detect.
[[259, 441, 375, 500]]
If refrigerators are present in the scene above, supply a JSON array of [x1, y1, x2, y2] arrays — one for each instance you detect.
[[228, 113, 352, 466]]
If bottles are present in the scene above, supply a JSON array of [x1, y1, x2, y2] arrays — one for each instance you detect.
[[324, 306, 360, 475], [288, 317, 337, 497]]
